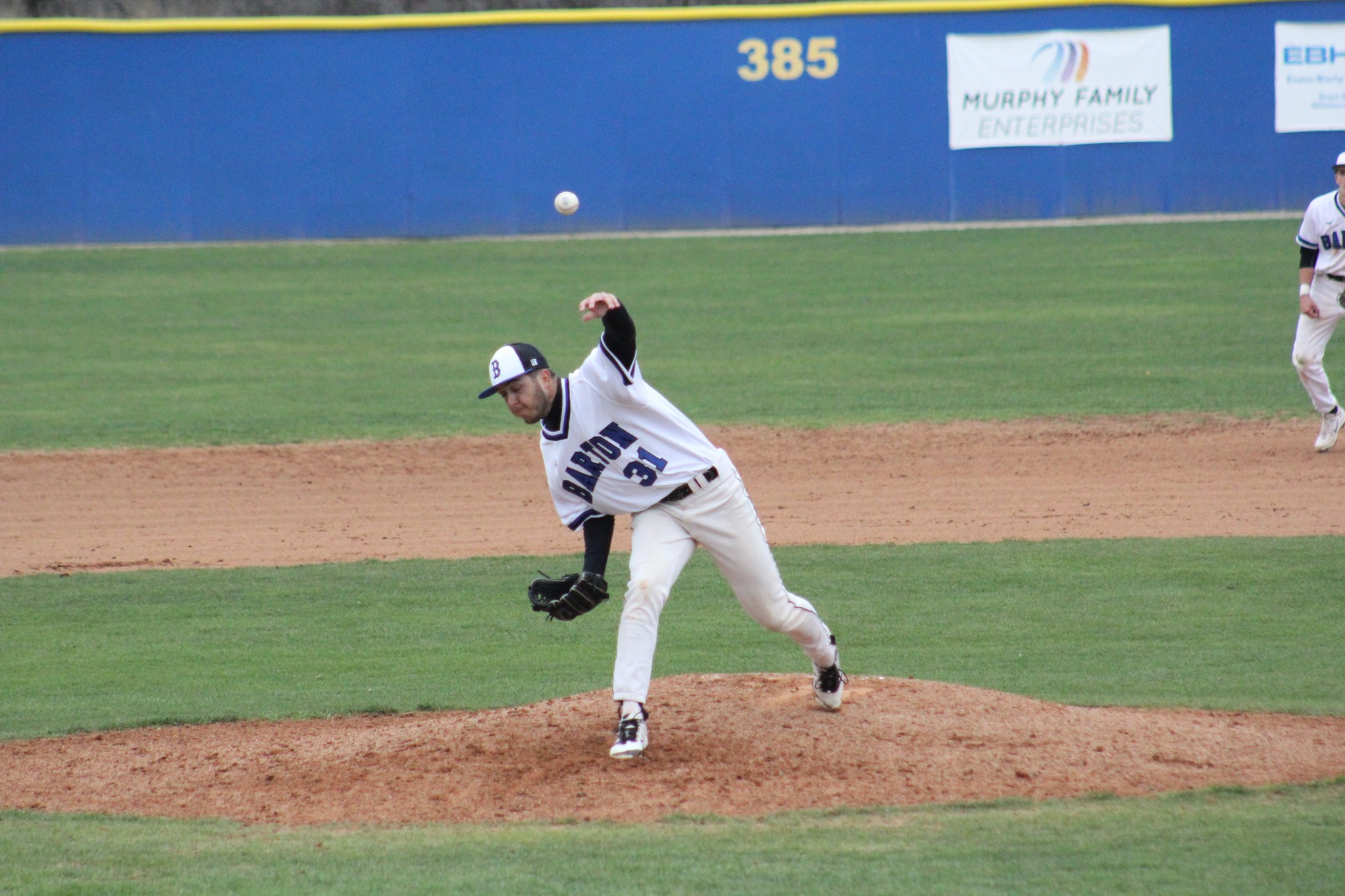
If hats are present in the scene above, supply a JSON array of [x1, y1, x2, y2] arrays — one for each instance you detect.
[[478, 342, 549, 399], [1332, 151, 1345, 168]]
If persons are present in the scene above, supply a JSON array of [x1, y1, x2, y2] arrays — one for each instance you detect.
[[1290, 150, 1345, 451], [476, 292, 850, 761]]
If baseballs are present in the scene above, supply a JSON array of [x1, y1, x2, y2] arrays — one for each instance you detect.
[[555, 191, 579, 215]]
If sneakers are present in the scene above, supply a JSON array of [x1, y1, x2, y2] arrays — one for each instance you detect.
[[812, 649, 848, 710], [1314, 405, 1345, 452], [610, 711, 648, 760]]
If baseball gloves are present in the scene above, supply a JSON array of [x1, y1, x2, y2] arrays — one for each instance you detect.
[[528, 571, 608, 621]]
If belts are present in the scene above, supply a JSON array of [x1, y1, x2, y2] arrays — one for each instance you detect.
[[660, 466, 718, 503], [1328, 273, 1345, 281]]
